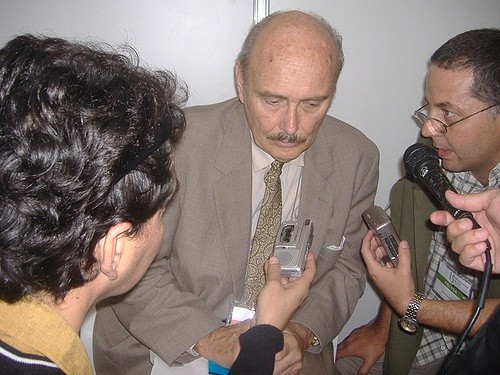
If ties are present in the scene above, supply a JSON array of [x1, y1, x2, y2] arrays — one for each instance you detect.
[[240, 160, 282, 308]]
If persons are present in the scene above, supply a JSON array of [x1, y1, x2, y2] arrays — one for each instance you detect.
[[0, 35, 318, 375], [92, 8, 380, 375], [361, 188, 500, 375], [336, 27, 500, 375]]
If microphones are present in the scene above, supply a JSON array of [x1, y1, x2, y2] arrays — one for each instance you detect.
[[403, 143, 492, 252]]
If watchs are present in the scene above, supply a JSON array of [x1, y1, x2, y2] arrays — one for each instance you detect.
[[399, 291, 426, 334], [310, 334, 319, 348]]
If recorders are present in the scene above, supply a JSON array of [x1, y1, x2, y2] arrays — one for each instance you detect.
[[362, 205, 401, 267], [273, 219, 314, 277]]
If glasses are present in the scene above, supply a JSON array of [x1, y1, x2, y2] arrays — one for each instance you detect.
[[414, 103, 498, 134]]
